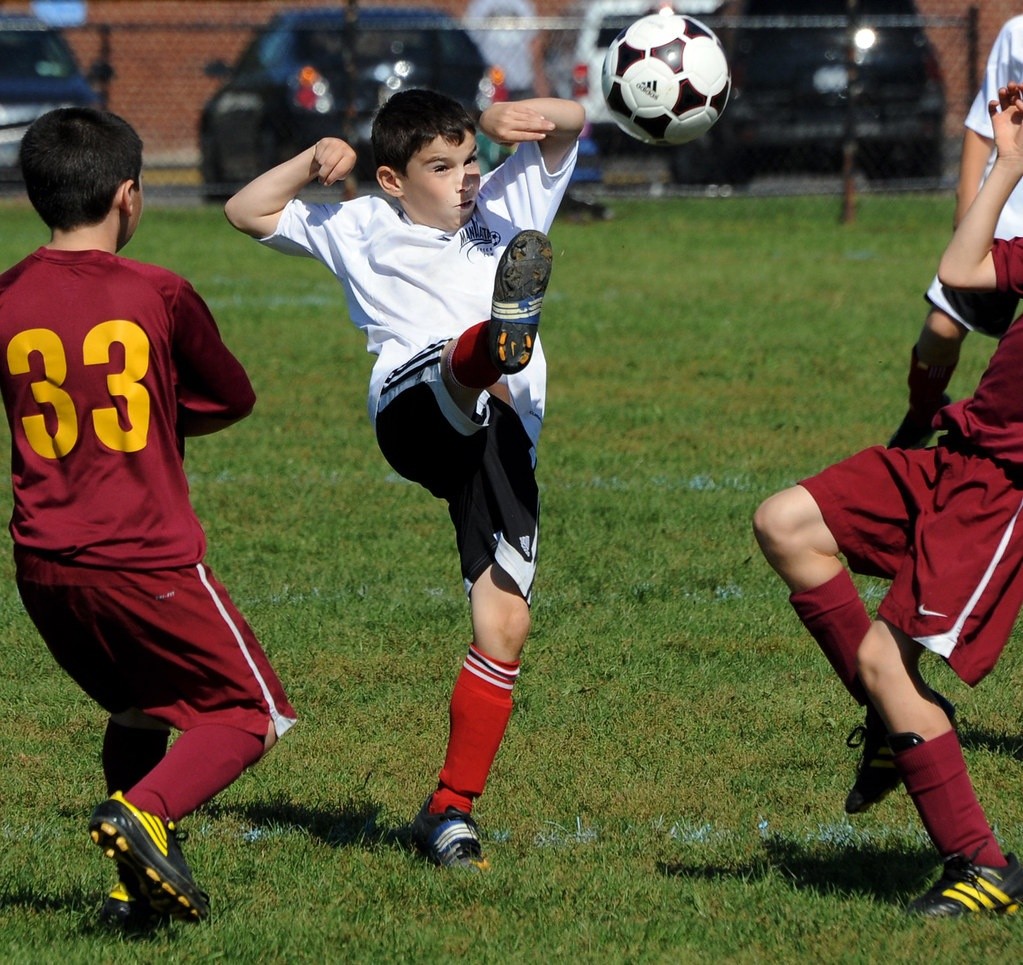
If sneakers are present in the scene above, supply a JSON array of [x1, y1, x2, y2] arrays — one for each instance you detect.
[[487, 230, 553, 375], [410, 795, 491, 871], [101, 882, 162, 933], [907, 851, 1023, 921], [845, 688, 956, 814], [88, 789, 211, 924]]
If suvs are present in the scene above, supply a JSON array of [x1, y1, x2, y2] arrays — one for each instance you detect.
[[671, 1, 949, 195], [542, 1, 727, 156]]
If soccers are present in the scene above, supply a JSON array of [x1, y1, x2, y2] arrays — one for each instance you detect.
[[599, 12, 733, 148]]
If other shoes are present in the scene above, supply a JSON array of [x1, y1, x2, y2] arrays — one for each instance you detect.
[[886, 406, 940, 451]]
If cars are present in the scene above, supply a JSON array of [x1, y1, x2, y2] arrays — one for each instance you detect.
[[197, 7, 511, 201], [0, 11, 103, 188]]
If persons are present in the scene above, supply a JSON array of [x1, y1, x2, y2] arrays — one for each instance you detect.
[[752, 12, 1023, 920], [223, 88, 585, 867], [0, 105, 300, 939]]
